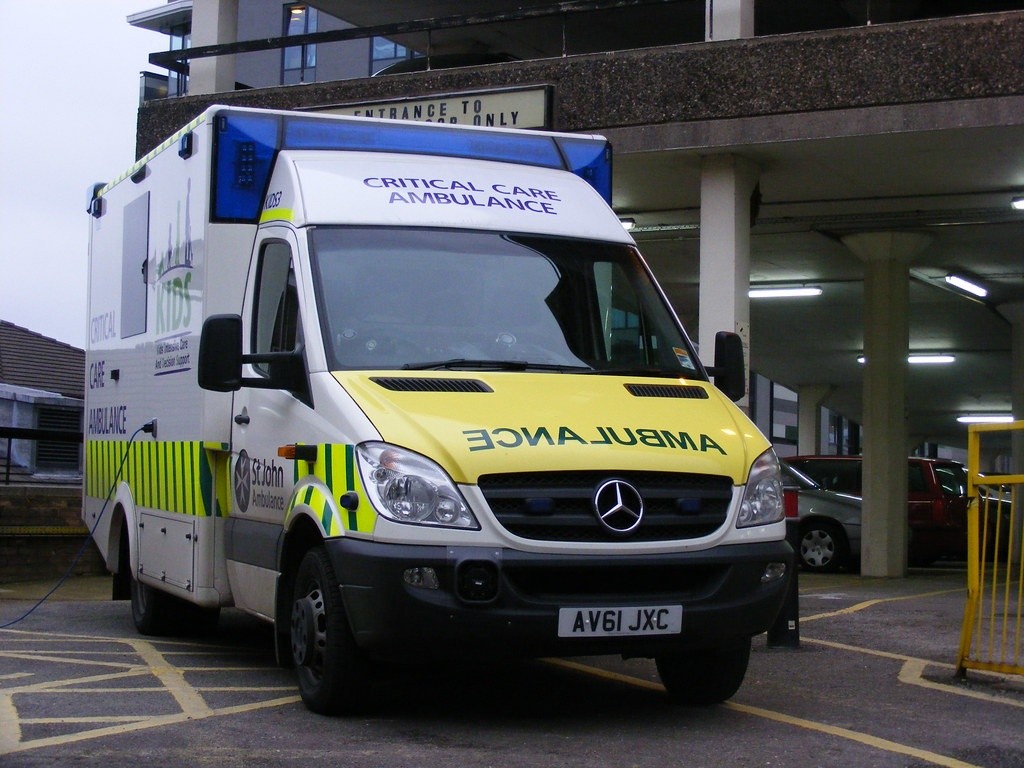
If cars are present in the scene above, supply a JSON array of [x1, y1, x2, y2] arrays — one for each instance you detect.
[[962, 466, 1012, 552], [778, 457, 913, 571]]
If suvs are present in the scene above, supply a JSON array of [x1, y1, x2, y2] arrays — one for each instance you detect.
[[778, 454, 984, 565]]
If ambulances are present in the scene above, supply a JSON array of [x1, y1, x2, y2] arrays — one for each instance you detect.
[[81, 103, 798, 718]]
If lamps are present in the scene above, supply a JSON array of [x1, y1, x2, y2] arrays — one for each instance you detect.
[[286, 5, 305, 15], [619, 218, 636, 230], [956, 413, 1014, 425], [748, 283, 823, 299], [944, 274, 988, 298], [855, 352, 956, 366], [1010, 196, 1024, 210]]
